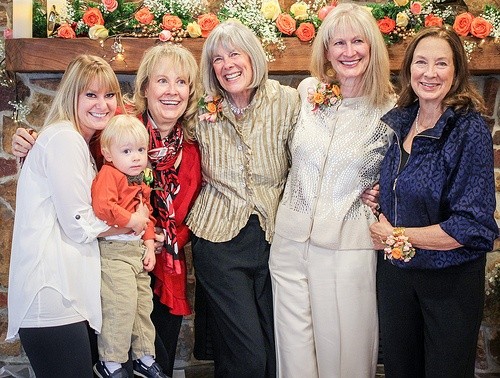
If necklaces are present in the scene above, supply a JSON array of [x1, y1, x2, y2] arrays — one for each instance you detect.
[[418, 113, 432, 129], [224, 91, 251, 114], [415, 107, 421, 133]]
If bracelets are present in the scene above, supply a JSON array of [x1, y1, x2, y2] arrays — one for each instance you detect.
[[384, 227, 415, 267]]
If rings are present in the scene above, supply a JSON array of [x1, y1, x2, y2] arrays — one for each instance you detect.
[[29, 130, 34, 135]]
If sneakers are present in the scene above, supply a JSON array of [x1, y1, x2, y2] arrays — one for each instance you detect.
[[93, 360, 130, 378], [133, 359, 169, 378]]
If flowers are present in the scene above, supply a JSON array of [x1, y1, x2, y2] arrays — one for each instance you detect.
[[306, 69, 343, 112], [4, 29, 12, 39], [55, 0, 500, 62], [144, 168, 164, 192], [198, 93, 224, 123]]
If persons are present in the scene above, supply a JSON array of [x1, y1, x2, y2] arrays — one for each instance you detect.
[[5, 2, 498, 378]]
